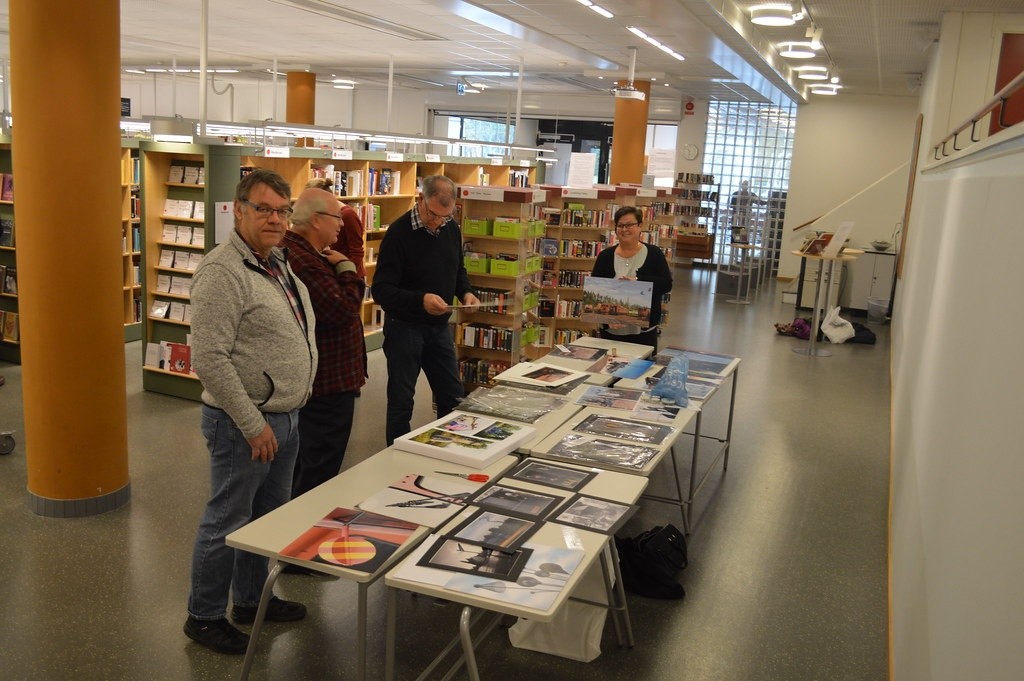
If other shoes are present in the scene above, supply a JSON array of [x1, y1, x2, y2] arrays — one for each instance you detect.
[[278, 559, 330, 579], [183, 612, 250, 655], [231, 595, 306, 625]]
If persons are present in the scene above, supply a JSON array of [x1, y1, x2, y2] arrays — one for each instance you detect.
[[371, 173, 480, 447], [730, 180, 768, 227], [588, 205, 672, 356], [275, 189, 369, 574], [306, 178, 365, 284], [183, 170, 318, 655]]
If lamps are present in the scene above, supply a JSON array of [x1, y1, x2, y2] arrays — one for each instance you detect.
[[752, 4, 839, 94]]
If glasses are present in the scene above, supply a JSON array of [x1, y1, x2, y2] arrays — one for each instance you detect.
[[238, 198, 293, 221], [423, 194, 453, 222], [614, 222, 638, 230], [314, 210, 343, 221]]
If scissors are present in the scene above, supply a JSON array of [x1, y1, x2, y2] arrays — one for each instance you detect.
[[434, 470, 489, 482]]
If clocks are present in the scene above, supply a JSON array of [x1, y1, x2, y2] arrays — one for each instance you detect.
[[682, 144, 699, 160]]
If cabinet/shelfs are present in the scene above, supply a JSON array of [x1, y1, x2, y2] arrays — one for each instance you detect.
[[841, 250, 897, 312], [0, 143, 721, 410]]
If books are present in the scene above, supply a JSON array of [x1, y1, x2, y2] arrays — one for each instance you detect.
[[460, 211, 541, 315], [800, 229, 833, 254], [312, 161, 425, 329], [0, 173, 20, 340], [605, 200, 675, 258], [479, 167, 529, 188], [122, 227, 127, 252], [529, 207, 609, 257], [534, 324, 589, 348], [674, 171, 714, 235], [453, 320, 540, 386], [129, 156, 143, 322], [537, 259, 592, 319], [143, 160, 209, 374]]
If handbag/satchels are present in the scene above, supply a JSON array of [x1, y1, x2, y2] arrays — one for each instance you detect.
[[774, 318, 824, 343], [614, 535, 684, 598], [633, 522, 688, 573], [824, 322, 877, 345], [820, 306, 855, 344], [508, 542, 620, 663]]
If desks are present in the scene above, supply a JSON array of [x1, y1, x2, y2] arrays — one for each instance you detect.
[[223, 337, 741, 681]]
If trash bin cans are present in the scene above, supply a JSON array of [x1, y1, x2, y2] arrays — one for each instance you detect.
[[867, 296, 890, 324]]
